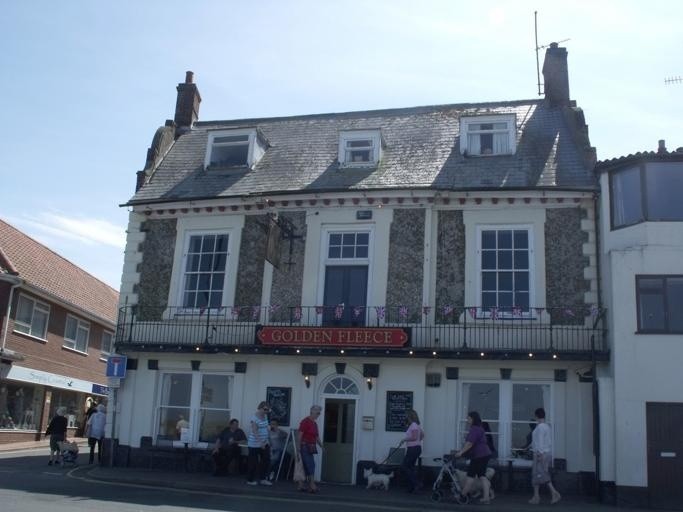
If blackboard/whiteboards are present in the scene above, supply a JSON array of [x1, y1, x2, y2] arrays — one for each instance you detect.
[[386, 391, 413, 431], [266, 387, 291, 426]]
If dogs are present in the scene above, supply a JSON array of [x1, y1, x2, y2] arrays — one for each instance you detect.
[[363, 467, 394, 493], [452, 467, 496, 505]]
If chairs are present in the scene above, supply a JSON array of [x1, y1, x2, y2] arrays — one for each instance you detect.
[[136, 435, 290, 491], [377, 448, 406, 484]]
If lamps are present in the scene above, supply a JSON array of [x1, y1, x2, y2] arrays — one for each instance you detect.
[[366, 378, 371, 390], [305, 375, 310, 388]]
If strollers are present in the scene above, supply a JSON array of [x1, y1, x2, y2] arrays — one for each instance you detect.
[[425, 453, 481, 506]]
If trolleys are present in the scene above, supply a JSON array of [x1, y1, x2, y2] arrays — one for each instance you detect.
[[57, 440, 79, 468]]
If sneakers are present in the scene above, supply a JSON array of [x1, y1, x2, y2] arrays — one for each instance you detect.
[[247, 480, 272, 485]]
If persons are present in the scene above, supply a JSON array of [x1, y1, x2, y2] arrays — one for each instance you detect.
[[174, 413, 190, 439], [523, 409, 563, 505], [482, 417, 538, 460], [43, 400, 106, 467], [208, 400, 327, 495], [454, 410, 493, 506], [398, 408, 425, 494], [471, 473, 497, 501]]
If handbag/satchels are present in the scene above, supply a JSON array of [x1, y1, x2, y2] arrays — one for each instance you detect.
[[532, 473, 550, 484], [469, 442, 481, 459], [293, 461, 305, 481], [57, 441, 78, 453], [307, 446, 316, 454]]
